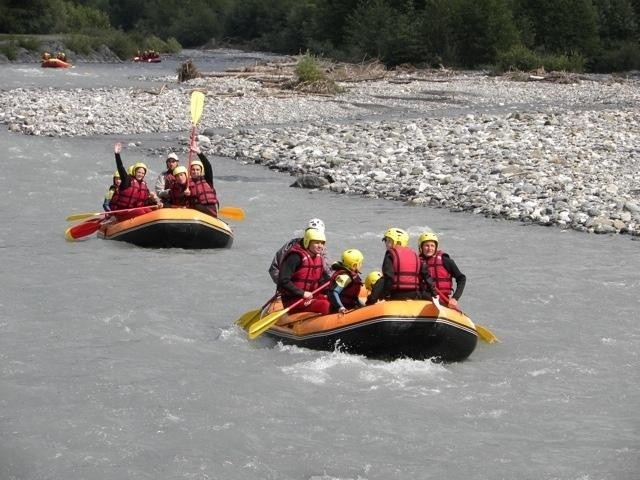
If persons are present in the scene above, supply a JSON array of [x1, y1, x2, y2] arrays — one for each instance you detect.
[[42, 50, 66, 61], [111, 142, 163, 222], [128, 165, 133, 174], [365, 228, 422, 306], [280, 229, 333, 314], [155, 153, 179, 198], [366, 271, 384, 292], [190, 143, 219, 218], [330, 249, 365, 313], [169, 166, 191, 207], [136, 49, 160, 60], [104, 170, 121, 212], [268, 217, 332, 284], [418, 232, 466, 308]]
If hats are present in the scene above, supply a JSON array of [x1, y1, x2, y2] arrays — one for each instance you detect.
[[164, 153, 179, 162]]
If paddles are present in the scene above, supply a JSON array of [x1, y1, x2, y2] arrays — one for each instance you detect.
[[435, 287, 502, 345], [69, 220, 112, 239], [186, 91, 204, 190], [248, 281, 330, 340], [64, 217, 107, 240], [233, 291, 280, 332], [65, 205, 158, 222], [217, 207, 245, 221]]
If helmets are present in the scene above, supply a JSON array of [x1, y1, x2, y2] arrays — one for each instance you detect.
[[303, 228, 327, 249], [304, 218, 326, 233], [385, 227, 409, 249], [132, 162, 148, 176], [191, 160, 204, 174], [340, 249, 364, 272], [127, 165, 134, 177], [364, 271, 383, 290], [112, 170, 120, 182], [172, 166, 189, 178], [418, 231, 439, 253]]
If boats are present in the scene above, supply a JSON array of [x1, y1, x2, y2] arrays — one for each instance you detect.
[[232, 295, 478, 364], [41, 58, 69, 67], [143, 58, 161, 63], [96, 207, 236, 249]]
